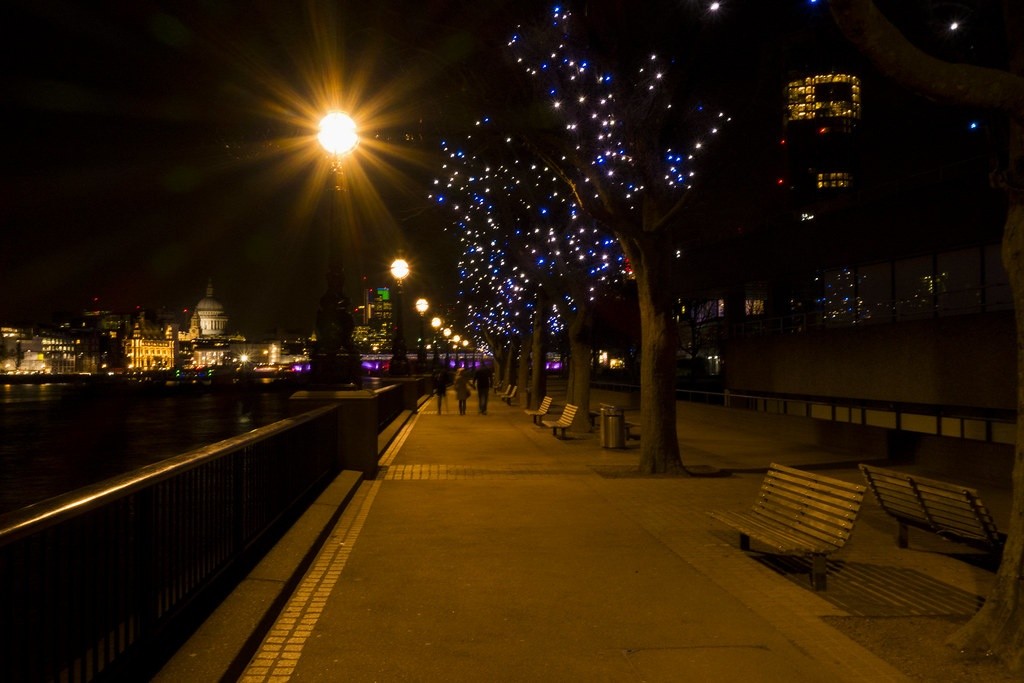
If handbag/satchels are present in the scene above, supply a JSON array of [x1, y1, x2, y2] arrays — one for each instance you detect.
[[466, 391, 470, 397]]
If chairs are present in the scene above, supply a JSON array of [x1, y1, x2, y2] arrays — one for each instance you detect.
[[497, 384, 518, 406], [523, 396, 552, 427]]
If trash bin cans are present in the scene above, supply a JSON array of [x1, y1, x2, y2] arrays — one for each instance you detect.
[[601, 408, 624, 448]]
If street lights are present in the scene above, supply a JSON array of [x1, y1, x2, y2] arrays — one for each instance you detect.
[[317, 108, 363, 390], [443, 328, 452, 365], [453, 335, 460, 362], [415, 298, 429, 373], [462, 339, 468, 359], [431, 317, 442, 369], [389, 259, 410, 375]]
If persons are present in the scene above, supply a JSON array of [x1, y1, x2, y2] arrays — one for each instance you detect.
[[472, 358, 494, 415], [432, 367, 449, 414], [455, 368, 476, 415]]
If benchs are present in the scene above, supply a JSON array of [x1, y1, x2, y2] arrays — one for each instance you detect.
[[703, 461, 1010, 593], [541, 404, 579, 440]]
